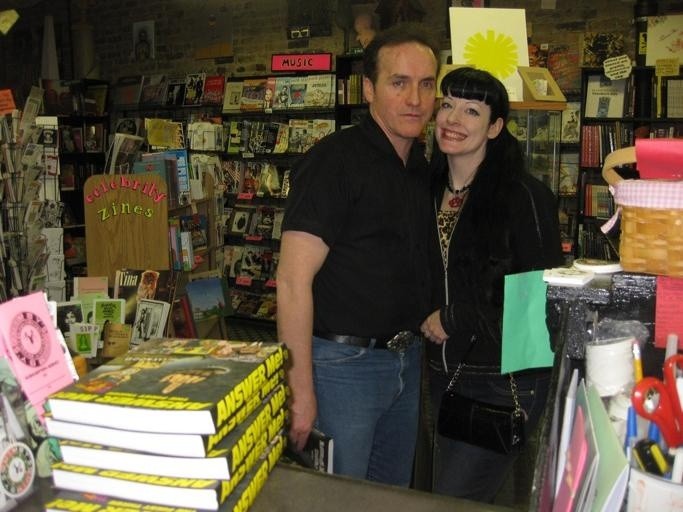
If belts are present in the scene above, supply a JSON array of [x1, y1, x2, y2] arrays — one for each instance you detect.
[[312, 329, 427, 351]]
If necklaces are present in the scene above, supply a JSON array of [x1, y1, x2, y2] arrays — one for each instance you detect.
[[447, 183, 472, 208]]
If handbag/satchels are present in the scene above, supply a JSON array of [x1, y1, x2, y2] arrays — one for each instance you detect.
[[437, 333, 526, 464]]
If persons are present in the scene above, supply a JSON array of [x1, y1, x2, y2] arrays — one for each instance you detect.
[[276, 22, 442, 487], [420, 67, 565, 504]]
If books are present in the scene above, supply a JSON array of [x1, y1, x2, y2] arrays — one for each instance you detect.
[[577, 75, 683, 262], [50, 73, 364, 330], [1, 91, 66, 300], [1, 270, 289, 512], [549, 357, 630, 512]]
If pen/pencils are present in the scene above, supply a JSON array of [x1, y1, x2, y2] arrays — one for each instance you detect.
[[622, 333, 683, 483]]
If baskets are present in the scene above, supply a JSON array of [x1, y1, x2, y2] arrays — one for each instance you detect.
[[602, 147, 683, 278]]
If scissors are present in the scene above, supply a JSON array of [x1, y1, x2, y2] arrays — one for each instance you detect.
[[632, 353, 683, 449]]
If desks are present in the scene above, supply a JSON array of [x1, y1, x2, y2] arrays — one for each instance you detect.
[[1, 463, 508, 512]]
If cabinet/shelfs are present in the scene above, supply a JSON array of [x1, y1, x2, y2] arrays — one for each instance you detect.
[[1, 55, 683, 323]]
